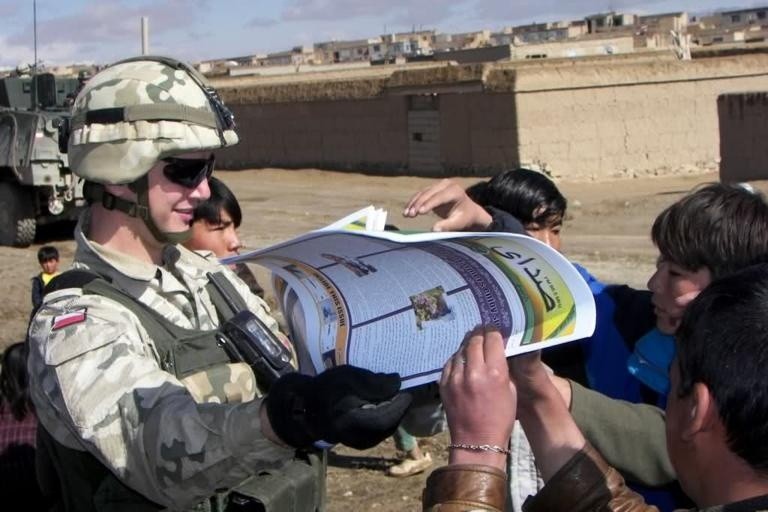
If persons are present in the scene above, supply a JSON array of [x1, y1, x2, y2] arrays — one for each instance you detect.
[[401, 169, 768, 511], [2, 56, 433, 512]]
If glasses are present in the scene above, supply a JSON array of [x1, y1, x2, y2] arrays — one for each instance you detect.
[[162, 154, 218, 188]]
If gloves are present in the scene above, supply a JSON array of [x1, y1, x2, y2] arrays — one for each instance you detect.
[[266, 362, 416, 451]]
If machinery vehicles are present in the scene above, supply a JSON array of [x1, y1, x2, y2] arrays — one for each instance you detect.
[[0, 0, 92, 247]]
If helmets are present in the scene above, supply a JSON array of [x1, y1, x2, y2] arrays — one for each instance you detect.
[[57, 53, 240, 186]]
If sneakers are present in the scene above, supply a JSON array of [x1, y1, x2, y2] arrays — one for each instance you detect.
[[388, 448, 434, 478]]
